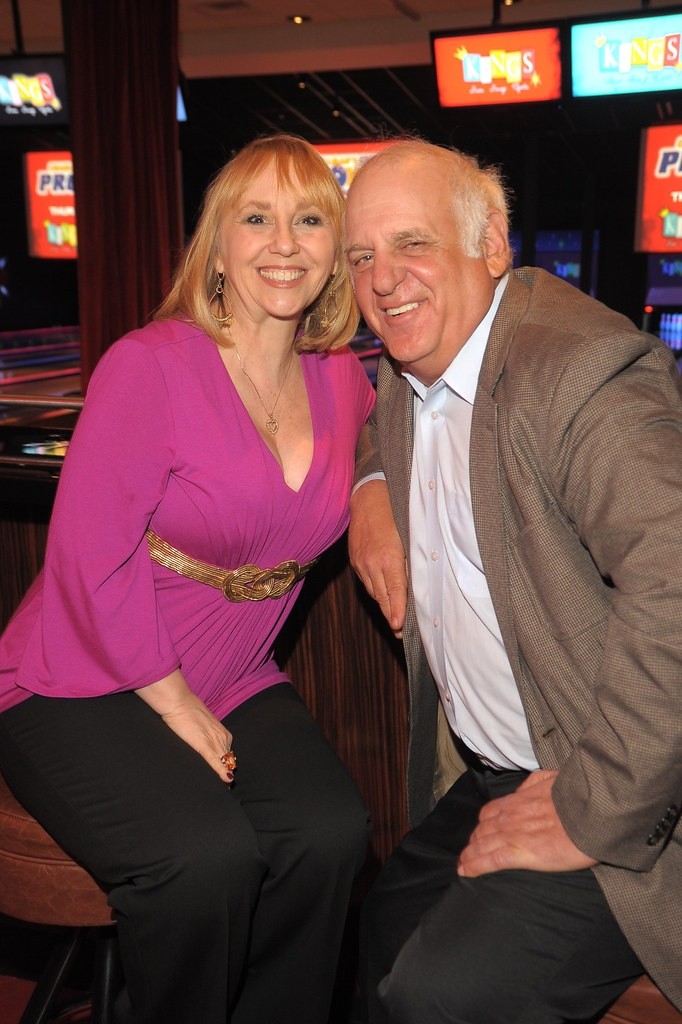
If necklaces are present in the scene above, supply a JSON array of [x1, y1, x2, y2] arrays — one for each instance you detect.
[[229, 330, 294, 435]]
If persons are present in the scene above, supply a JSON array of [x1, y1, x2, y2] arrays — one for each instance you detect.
[[345, 139, 682, 1024], [0, 135, 374, 1023]]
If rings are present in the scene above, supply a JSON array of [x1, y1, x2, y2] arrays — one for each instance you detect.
[[220, 751, 237, 771]]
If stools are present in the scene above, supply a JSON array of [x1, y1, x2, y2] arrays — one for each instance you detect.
[[0, 781, 117, 1024]]
